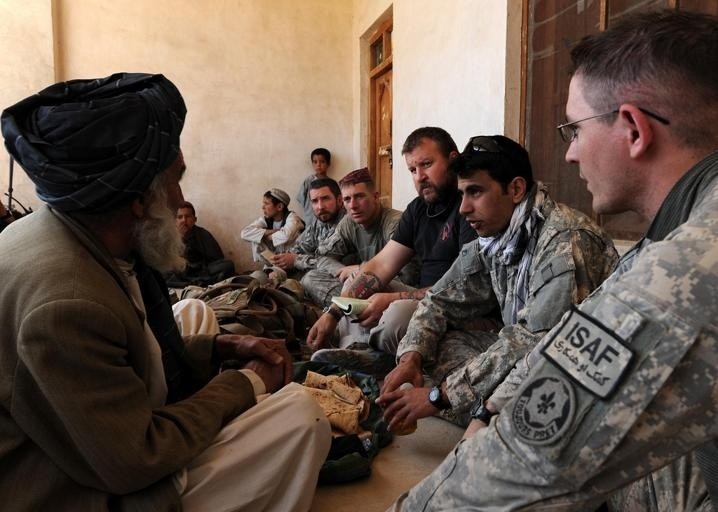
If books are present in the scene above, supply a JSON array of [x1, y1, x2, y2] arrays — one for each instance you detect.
[[329, 294, 371, 316]]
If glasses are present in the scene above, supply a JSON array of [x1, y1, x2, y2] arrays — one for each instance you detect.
[[556, 105, 673, 145]]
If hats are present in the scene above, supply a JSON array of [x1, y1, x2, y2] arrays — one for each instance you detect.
[[263, 188, 290, 207], [338, 166, 373, 184], [461, 134, 530, 165], [1, 72, 188, 217]]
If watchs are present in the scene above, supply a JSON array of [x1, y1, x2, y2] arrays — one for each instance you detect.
[[427, 386, 453, 414], [322, 307, 342, 323], [469, 397, 492, 426]]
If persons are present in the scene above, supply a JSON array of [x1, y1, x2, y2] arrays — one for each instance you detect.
[[305, 125, 480, 371], [0, 72, 331, 512], [294, 145, 333, 230], [271, 177, 348, 280], [169, 199, 227, 286], [239, 188, 306, 280], [384, 6, 718, 512], [297, 168, 404, 309], [375, 133, 623, 430]]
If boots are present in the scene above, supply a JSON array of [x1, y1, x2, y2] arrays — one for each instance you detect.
[[308, 347, 396, 381], [419, 373, 472, 427]]
